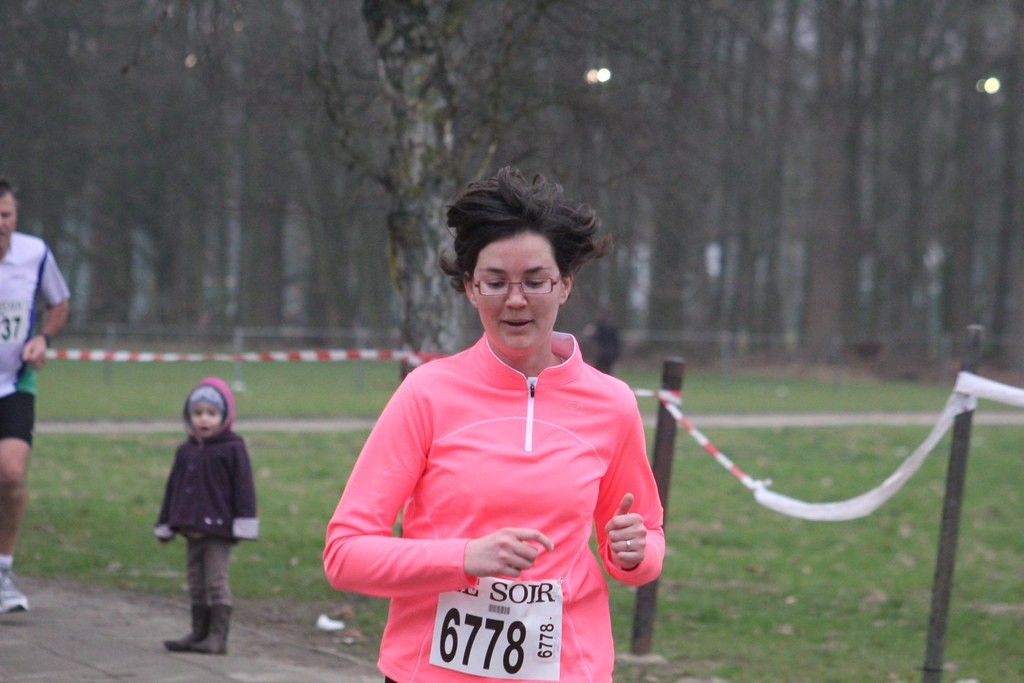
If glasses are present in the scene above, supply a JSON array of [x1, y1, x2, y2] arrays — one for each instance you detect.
[[474, 275, 558, 296]]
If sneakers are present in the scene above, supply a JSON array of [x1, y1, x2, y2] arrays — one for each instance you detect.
[[0, 568, 30, 612]]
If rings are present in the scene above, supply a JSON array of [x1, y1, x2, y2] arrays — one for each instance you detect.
[[626, 542, 632, 553]]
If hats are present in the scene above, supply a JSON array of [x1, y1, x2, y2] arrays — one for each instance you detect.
[[190, 386, 227, 411]]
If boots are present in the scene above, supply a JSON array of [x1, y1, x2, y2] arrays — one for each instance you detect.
[[187, 604, 232, 654], [163, 603, 212, 650]]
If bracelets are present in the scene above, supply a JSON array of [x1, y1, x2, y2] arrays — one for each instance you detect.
[[39, 332, 52, 346]]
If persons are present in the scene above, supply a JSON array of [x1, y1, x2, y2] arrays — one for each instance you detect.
[[323, 166, 667, 683], [0, 180, 71, 615], [154, 375, 258, 657], [580, 301, 621, 370]]
